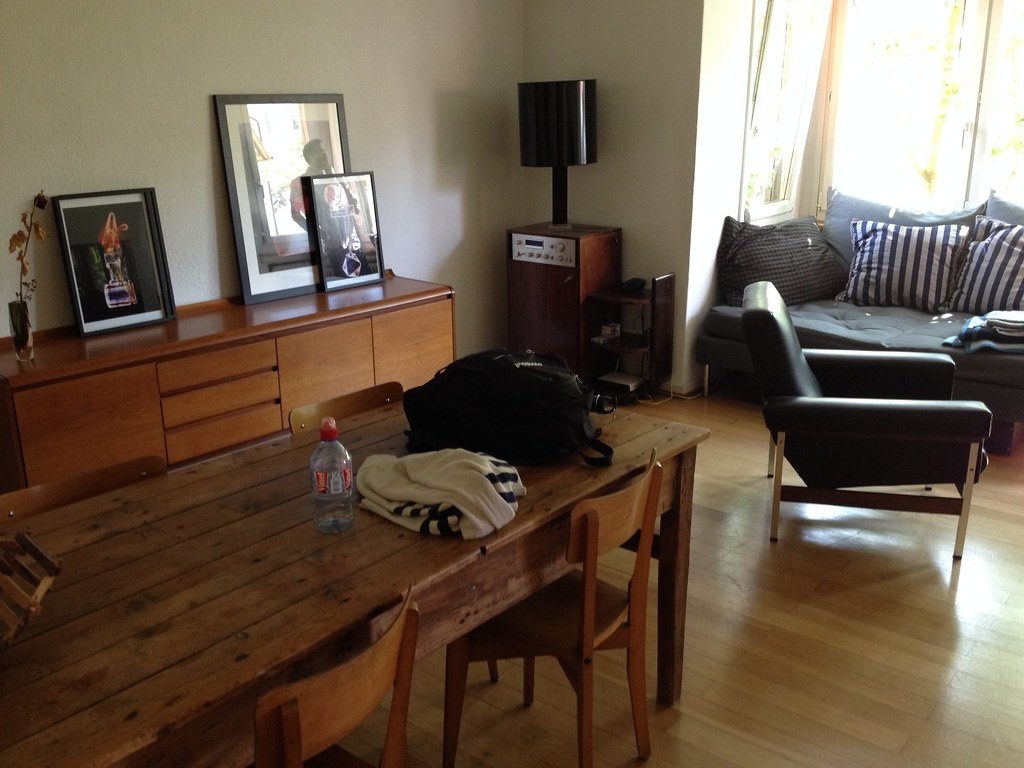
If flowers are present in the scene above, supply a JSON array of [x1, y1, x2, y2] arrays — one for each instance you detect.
[[9, 188, 47, 300]]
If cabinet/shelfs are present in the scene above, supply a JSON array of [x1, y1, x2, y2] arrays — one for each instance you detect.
[[505, 223, 622, 389], [583, 273, 676, 401], [0, 271, 456, 487]]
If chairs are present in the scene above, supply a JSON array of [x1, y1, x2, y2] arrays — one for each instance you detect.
[[1, 458, 165, 533], [288, 381, 404, 441], [253, 583, 419, 768], [741, 280, 993, 563], [439, 449, 663, 768]]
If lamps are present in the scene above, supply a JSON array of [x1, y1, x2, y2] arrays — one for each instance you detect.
[[518, 78, 594, 223]]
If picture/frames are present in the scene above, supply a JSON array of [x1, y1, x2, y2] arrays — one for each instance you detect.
[[303, 175, 386, 294], [49, 188, 176, 345], [213, 92, 353, 305]]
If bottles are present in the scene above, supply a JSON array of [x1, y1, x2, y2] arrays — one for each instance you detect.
[[309, 417, 355, 533]]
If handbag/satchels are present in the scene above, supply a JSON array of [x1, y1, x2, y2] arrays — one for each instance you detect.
[[400, 347, 614, 471]]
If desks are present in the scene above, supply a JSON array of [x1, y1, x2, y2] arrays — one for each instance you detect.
[[0, 394, 710, 768]]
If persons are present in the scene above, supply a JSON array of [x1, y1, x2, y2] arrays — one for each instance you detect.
[[314, 183, 369, 276], [290, 138, 334, 232]]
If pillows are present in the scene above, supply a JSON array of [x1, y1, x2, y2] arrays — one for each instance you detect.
[[934, 214, 1024, 313], [985, 188, 1024, 226], [835, 219, 970, 313], [822, 186, 988, 262], [716, 215, 851, 306]]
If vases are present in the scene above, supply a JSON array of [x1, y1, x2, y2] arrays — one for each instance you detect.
[[9, 302, 33, 361]]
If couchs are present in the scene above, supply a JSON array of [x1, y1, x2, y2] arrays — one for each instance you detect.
[[694, 221, 1024, 456]]
